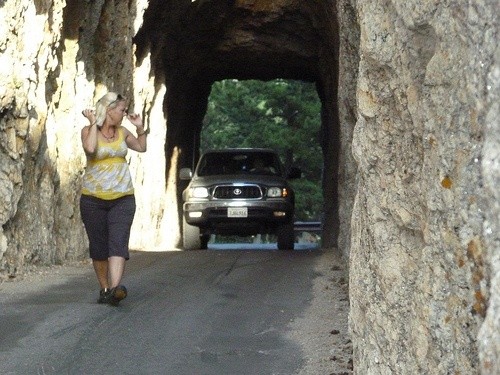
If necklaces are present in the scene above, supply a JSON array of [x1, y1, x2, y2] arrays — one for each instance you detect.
[[97, 126, 115, 143]]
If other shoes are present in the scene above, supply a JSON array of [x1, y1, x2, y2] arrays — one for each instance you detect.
[[97, 284, 128, 306]]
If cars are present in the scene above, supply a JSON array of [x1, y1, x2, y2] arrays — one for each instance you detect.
[[180, 147, 302, 250]]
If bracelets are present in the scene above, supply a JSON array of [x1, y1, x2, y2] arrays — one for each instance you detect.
[[136, 129, 144, 135], [89, 120, 97, 129]]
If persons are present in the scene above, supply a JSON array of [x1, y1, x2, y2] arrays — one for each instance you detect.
[[249, 156, 276, 174], [79, 92, 147, 306]]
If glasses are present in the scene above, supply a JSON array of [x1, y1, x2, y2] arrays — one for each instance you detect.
[[108, 94, 126, 108]]
